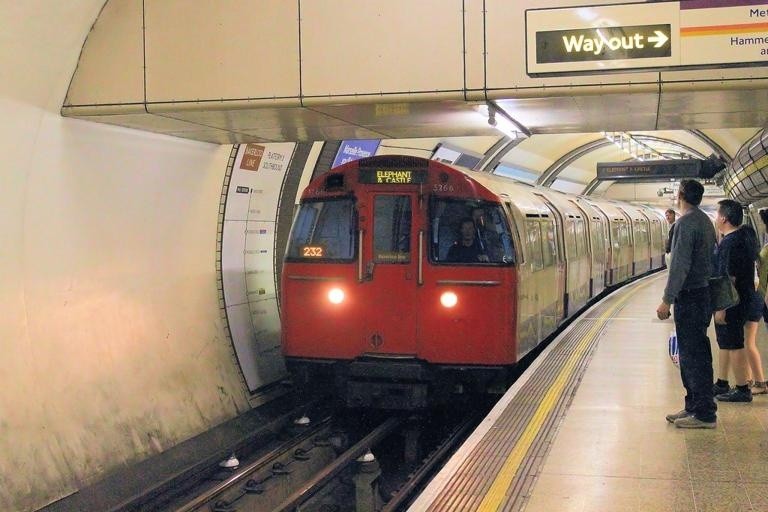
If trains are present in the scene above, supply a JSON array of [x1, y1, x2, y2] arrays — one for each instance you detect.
[[280, 154, 718, 413]]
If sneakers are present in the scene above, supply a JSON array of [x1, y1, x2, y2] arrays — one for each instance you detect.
[[712, 382, 730, 396], [666, 409, 695, 422], [674, 417, 717, 429], [716, 388, 752, 402]]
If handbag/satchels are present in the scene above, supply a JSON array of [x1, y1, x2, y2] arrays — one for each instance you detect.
[[709, 275, 740, 311]]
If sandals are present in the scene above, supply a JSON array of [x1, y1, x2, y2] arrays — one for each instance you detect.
[[749, 381, 768, 395]]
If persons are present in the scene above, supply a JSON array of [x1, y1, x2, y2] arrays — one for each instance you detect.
[[737, 224, 768, 397], [654, 178, 719, 430], [663, 209, 677, 276], [444, 217, 493, 263], [711, 199, 754, 403], [749, 208, 768, 329]]
